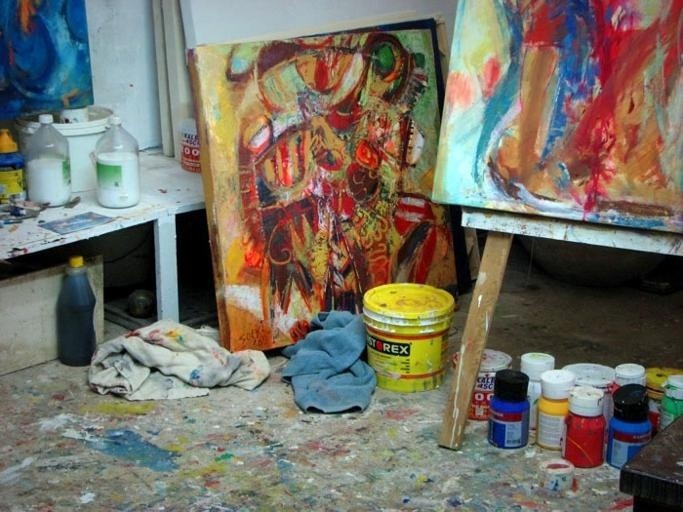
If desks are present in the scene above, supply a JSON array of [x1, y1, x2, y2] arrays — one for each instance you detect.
[[0, 147, 205, 324]]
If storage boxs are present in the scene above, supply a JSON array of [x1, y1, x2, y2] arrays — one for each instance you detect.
[[0, 249, 104, 376]]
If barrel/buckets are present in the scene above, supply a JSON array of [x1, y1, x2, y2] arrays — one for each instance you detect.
[[180, 116, 201, 174], [560, 361, 616, 444], [451, 347, 512, 424], [15, 105, 117, 194], [363, 282, 456, 396]]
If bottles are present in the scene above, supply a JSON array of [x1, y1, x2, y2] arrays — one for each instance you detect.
[[491, 352, 683, 472], [1, 115, 140, 211]]
